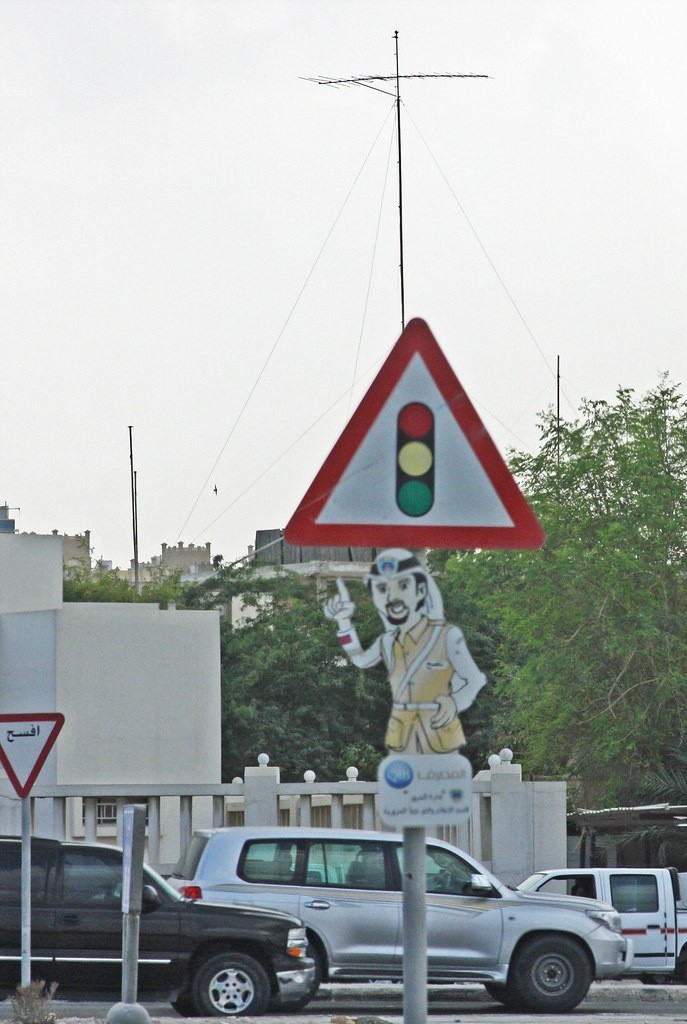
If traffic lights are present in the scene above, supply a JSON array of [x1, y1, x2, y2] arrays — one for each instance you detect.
[[395, 400, 436, 519]]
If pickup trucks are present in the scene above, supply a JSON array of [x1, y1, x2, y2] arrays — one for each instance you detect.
[[512, 867, 687, 986]]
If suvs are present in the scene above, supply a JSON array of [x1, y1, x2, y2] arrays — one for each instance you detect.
[[0, 832, 316, 1017], [165, 824, 635, 1016]]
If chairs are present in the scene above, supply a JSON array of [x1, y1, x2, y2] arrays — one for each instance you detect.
[[276, 850, 294, 881]]
